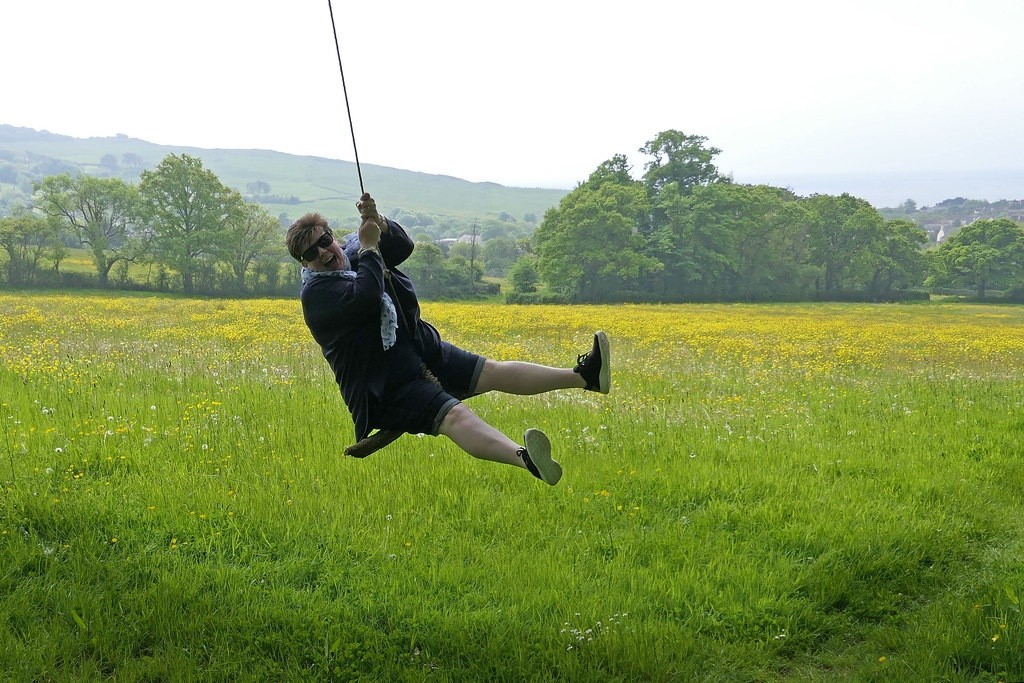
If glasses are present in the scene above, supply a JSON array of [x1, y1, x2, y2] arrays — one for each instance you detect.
[[300, 231, 334, 262]]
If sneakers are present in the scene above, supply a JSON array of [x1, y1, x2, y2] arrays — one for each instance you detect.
[[516, 428, 562, 486], [573, 331, 611, 394]]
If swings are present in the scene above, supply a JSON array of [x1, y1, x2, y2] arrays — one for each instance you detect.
[[325, 0, 413, 459]]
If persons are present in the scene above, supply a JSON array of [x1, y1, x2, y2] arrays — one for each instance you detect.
[[286, 193, 611, 486]]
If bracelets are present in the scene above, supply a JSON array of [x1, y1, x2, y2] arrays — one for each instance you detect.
[[377, 213, 385, 225], [358, 245, 377, 254]]
